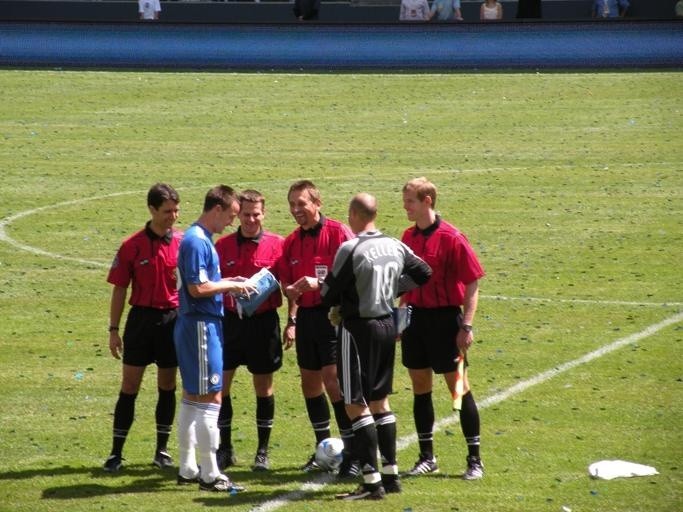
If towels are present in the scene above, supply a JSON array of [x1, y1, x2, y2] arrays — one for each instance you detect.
[[588, 459, 659, 480]]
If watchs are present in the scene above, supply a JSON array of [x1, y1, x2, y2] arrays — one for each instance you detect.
[[460, 322, 474, 332], [317, 276, 325, 289]]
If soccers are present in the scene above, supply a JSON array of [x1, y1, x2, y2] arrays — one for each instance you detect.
[[315, 438, 345, 470]]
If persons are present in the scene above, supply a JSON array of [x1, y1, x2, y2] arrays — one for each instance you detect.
[[426, 0, 464, 22], [293, 1, 321, 21], [279, 179, 363, 486], [173, 184, 260, 494], [319, 192, 433, 502], [101, 183, 184, 472], [215, 189, 298, 473], [592, 0, 629, 20], [138, 0, 164, 20], [480, 0, 503, 21], [676, 1, 683, 22], [395, 177, 485, 481], [399, 0, 431, 22]]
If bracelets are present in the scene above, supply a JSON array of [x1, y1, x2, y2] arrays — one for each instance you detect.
[[108, 325, 120, 333], [287, 315, 298, 326]]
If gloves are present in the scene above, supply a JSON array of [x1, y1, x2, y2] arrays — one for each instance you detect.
[[328, 305, 342, 325]]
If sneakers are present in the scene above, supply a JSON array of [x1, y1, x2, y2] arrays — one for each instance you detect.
[[103, 455, 126, 471], [216, 453, 233, 469], [334, 479, 386, 500], [254, 448, 268, 470], [199, 474, 246, 492], [462, 455, 484, 480], [404, 453, 439, 476], [177, 465, 201, 485], [336, 460, 360, 479], [381, 473, 401, 493], [302, 453, 319, 471], [152, 451, 175, 468]]
